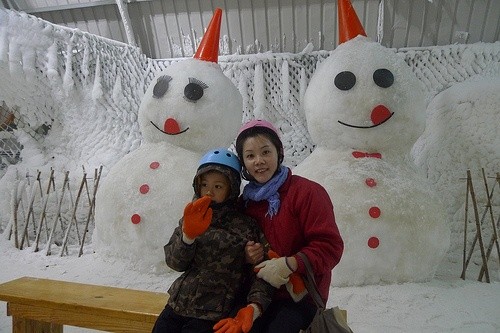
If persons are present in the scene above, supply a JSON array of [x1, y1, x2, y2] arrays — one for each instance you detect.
[[152, 148, 274, 333], [234, 119, 344, 333]]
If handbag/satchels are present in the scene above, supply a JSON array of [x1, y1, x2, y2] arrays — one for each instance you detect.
[[298, 252, 355, 333]]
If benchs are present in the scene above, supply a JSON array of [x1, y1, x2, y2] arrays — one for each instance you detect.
[[0, 277, 346, 333]]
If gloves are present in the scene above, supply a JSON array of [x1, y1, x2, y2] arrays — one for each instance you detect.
[[254, 255, 295, 289], [266, 249, 308, 302], [212, 302, 262, 333], [182, 196, 213, 245]]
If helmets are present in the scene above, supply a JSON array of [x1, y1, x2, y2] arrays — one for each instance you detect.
[[236, 119, 284, 170], [196, 147, 242, 174]]
